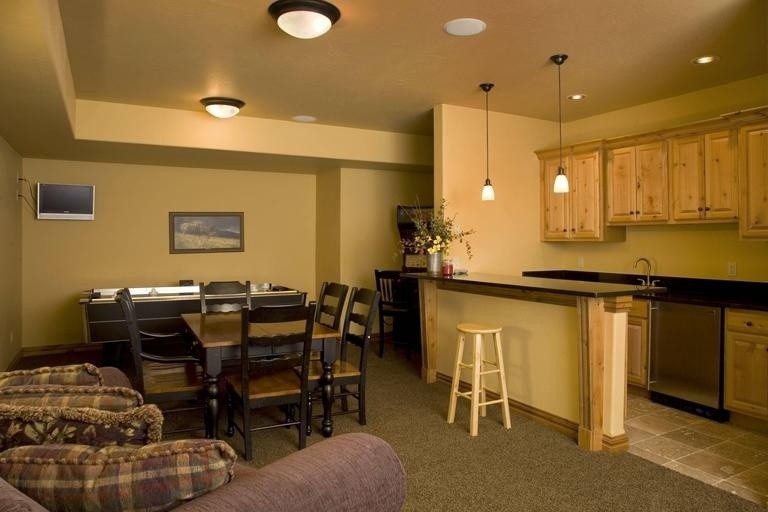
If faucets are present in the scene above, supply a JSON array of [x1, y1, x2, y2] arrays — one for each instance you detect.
[[634, 258, 651, 285]]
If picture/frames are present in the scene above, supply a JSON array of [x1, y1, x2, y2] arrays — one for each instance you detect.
[[169, 212, 244, 254]]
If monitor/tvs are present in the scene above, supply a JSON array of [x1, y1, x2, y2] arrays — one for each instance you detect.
[[36, 182, 96, 220]]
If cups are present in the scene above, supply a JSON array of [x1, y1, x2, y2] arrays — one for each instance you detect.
[[440, 259, 454, 277]]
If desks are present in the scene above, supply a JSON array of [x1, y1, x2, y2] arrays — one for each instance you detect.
[[78, 292, 309, 374]]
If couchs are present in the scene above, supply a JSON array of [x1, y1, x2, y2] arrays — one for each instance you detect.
[[0, 367, 407, 512]]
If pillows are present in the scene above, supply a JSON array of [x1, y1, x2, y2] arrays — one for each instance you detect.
[[0, 438, 237, 512], [0, 403, 165, 452], [0, 362, 104, 386], [0, 384, 143, 411]]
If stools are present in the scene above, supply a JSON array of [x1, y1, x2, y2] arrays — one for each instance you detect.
[[447, 322, 511, 436]]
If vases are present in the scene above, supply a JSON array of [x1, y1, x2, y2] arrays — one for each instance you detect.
[[426, 253, 442, 277]]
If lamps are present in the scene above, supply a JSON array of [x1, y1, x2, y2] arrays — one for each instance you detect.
[[267, 0, 341, 39], [200, 97, 245, 118], [479, 83, 496, 201], [550, 54, 570, 193]]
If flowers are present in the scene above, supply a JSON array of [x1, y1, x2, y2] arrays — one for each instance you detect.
[[391, 192, 477, 263]]
